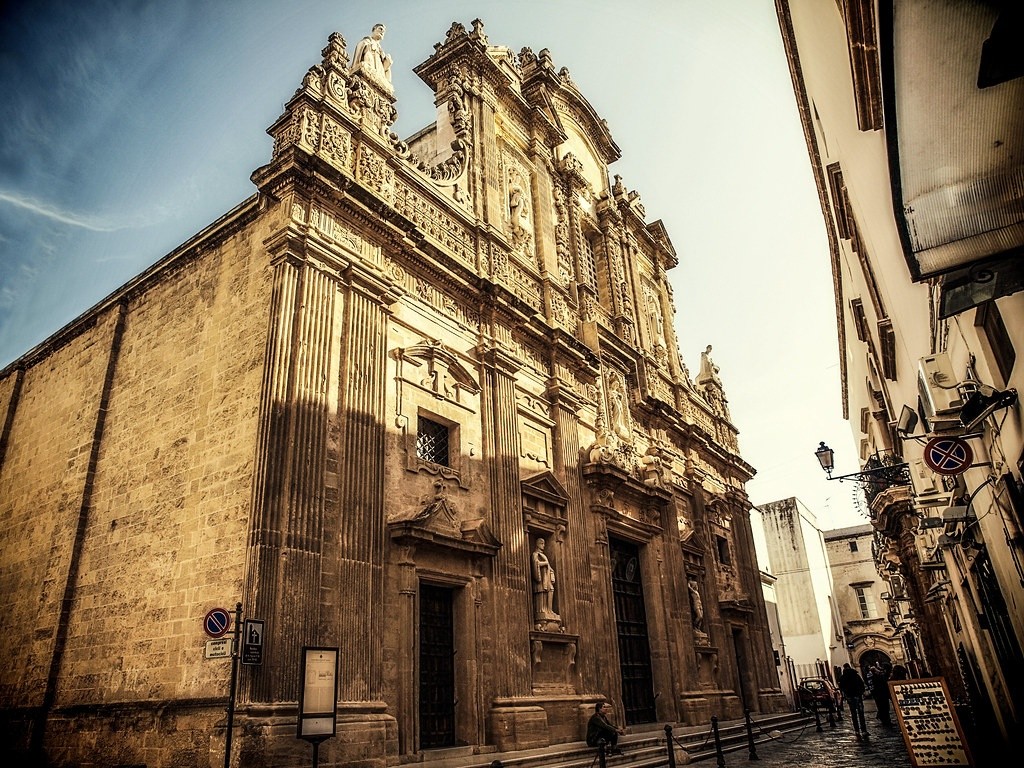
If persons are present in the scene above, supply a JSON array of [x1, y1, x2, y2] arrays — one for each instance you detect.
[[648, 296, 663, 337], [861, 658, 907, 728], [531, 537, 556, 613], [586, 702, 626, 757], [352, 23, 393, 83], [688, 581, 704, 632], [700, 344, 721, 374], [838, 662, 871, 737], [509, 175, 528, 220], [610, 381, 623, 424]]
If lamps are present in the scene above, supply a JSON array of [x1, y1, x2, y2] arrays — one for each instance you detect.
[[884, 561, 904, 572], [814, 441, 913, 488], [880, 591, 910, 601]]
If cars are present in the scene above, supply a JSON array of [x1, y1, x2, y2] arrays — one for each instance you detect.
[[801, 679, 844, 712]]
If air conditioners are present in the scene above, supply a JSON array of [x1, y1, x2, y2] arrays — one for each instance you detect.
[[908, 458, 936, 497], [917, 353, 964, 432], [889, 576, 904, 599], [914, 534, 940, 564]]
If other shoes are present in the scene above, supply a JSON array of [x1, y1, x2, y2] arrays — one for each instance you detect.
[[862, 732, 871, 736], [855, 731, 859, 735], [604, 750, 612, 757], [611, 749, 623, 755]]
